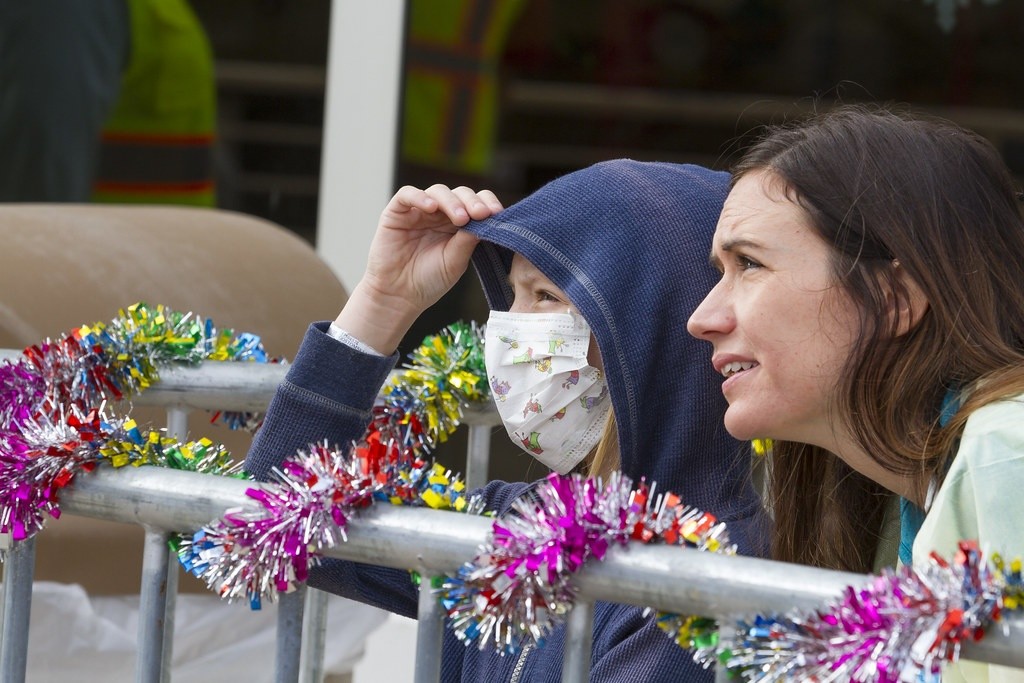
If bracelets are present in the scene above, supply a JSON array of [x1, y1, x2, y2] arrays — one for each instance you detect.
[[325, 324, 384, 357]]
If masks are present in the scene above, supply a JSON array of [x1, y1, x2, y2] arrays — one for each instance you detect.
[[484, 310, 612, 475]]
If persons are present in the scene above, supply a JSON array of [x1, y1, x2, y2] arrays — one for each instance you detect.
[[246, 156, 775, 683], [686, 113, 1024, 683], [397, 0, 550, 182], [0, 0, 217, 205]]
